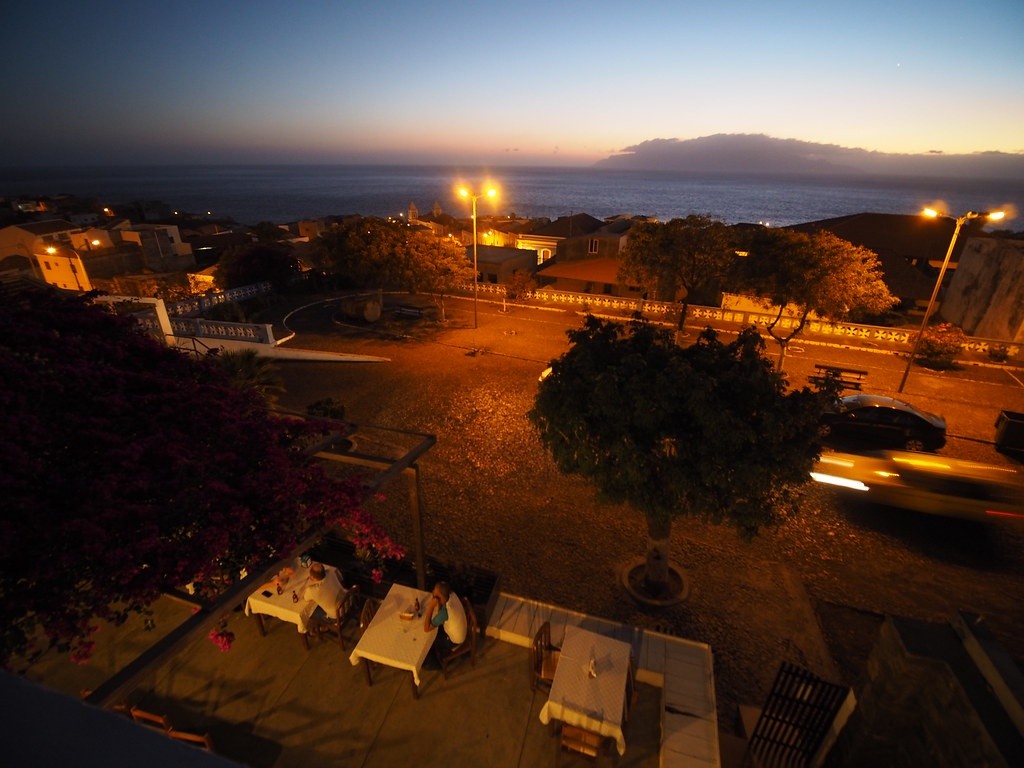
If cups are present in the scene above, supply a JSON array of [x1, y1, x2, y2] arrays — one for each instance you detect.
[[418, 611, 422, 617]]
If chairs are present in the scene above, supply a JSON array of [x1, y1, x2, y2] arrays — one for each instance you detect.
[[441, 597, 479, 678], [530, 622, 561, 691], [315, 585, 359, 651], [360, 600, 375, 636]]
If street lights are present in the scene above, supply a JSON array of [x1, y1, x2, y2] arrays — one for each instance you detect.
[[46, 239, 100, 290], [459, 187, 497, 328], [898, 206, 1004, 393]]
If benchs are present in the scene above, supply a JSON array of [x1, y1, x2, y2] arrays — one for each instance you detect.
[[808, 364, 868, 391]]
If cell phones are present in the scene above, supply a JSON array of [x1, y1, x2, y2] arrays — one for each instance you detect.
[[262, 591, 272, 597]]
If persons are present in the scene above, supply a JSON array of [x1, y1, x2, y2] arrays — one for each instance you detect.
[[297, 564, 346, 647], [422, 581, 467, 671]]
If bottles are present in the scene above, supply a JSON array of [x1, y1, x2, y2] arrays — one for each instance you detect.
[[415, 598, 420, 611], [277, 583, 283, 595], [292, 590, 298, 603]]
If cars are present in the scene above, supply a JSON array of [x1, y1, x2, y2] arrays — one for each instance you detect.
[[815, 395, 947, 452]]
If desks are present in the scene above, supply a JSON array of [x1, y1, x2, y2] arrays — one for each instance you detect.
[[245, 561, 343, 651], [539, 626, 634, 757], [349, 582, 438, 700]]
